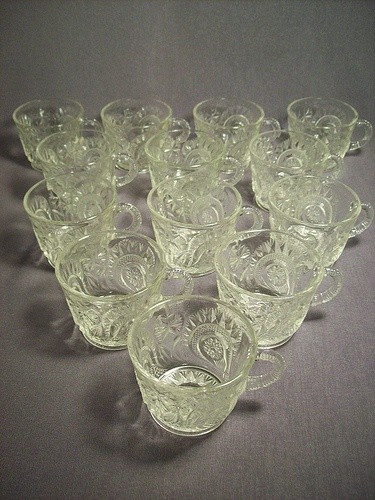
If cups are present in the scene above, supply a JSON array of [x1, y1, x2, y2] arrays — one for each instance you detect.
[[147, 173, 264, 279], [194, 96, 280, 186], [286, 96, 374, 160], [101, 96, 190, 174], [36, 129, 138, 188], [127, 295, 286, 437], [268, 174, 374, 268], [55, 229, 194, 351], [214, 228, 344, 350], [249, 129, 345, 212], [145, 128, 244, 187], [12, 97, 103, 171], [23, 171, 142, 269]]
